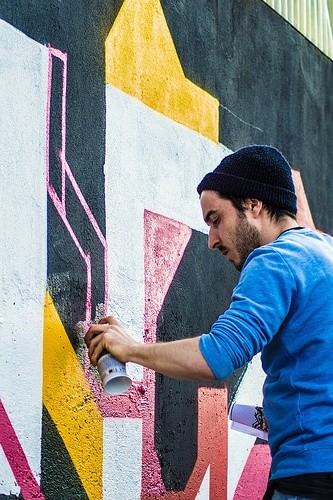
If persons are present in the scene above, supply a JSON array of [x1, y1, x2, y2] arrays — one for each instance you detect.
[[84, 145, 333, 500]]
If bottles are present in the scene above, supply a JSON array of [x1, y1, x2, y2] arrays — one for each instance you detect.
[[96, 352, 132, 395]]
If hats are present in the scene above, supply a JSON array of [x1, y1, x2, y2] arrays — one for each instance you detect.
[[197, 145, 300, 215]]
